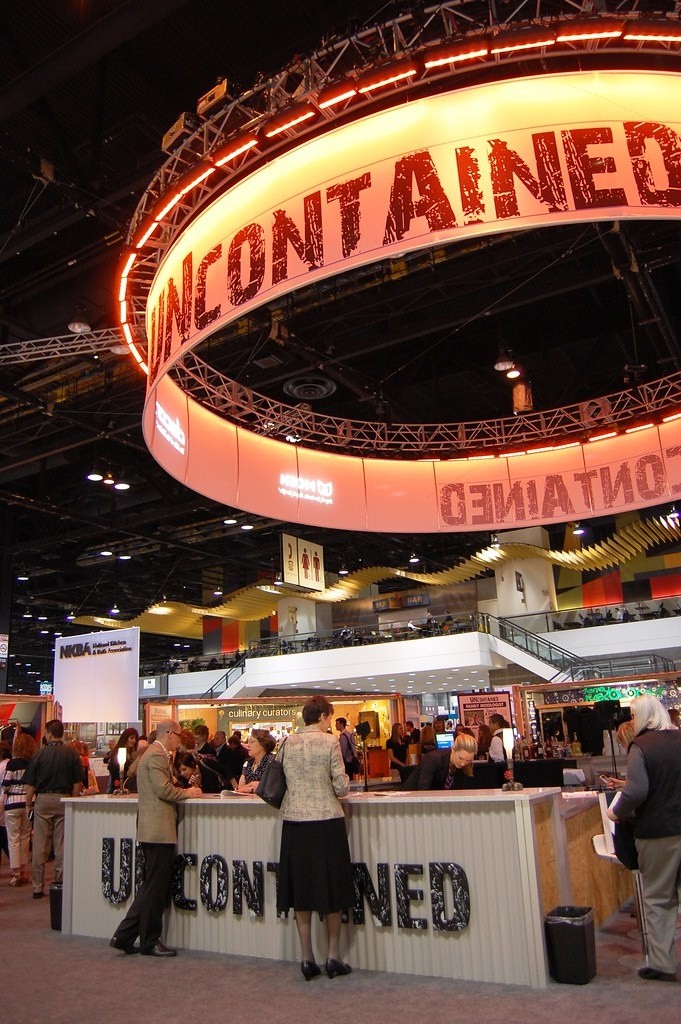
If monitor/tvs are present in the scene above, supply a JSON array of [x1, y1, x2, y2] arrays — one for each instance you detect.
[[434, 733, 456, 749]]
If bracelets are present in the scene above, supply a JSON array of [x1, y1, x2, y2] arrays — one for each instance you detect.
[[250, 787, 253, 793]]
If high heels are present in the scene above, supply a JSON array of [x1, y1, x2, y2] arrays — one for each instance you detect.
[[325, 957, 352, 979], [300, 960, 322, 982]]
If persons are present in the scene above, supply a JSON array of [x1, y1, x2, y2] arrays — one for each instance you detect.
[[340, 625, 352, 646], [276, 696, 352, 980], [407, 610, 454, 638], [167, 648, 247, 671], [595, 605, 625, 622], [606, 695, 681, 982], [0, 718, 91, 899], [102, 712, 511, 794], [109, 718, 202, 958]]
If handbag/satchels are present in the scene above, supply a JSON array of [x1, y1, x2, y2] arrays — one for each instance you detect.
[[256, 737, 288, 809], [344, 757, 360, 774], [79, 769, 99, 795]]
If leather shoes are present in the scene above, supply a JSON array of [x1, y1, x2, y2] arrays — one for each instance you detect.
[[109, 936, 140, 954], [141, 942, 177, 957]]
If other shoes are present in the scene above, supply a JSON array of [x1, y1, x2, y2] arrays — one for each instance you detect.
[[33, 891, 45, 899], [638, 967, 676, 982], [7, 870, 28, 887]]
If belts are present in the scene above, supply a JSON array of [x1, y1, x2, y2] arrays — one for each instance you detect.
[[41, 790, 67, 794]]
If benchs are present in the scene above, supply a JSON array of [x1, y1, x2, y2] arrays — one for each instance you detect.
[[553, 609, 681, 630]]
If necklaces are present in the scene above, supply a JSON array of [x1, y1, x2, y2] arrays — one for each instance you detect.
[[252, 759, 256, 770]]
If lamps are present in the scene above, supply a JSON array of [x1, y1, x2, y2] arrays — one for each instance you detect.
[[339, 560, 349, 575], [490, 535, 500, 548], [214, 586, 222, 595], [110, 602, 120, 614], [572, 521, 584, 534], [100, 540, 132, 559], [492, 346, 525, 381], [17, 566, 29, 580], [669, 505, 678, 518], [68, 308, 92, 334], [174, 637, 181, 646], [22, 606, 76, 635], [15, 655, 32, 666], [224, 508, 254, 530], [409, 551, 420, 562], [86, 454, 132, 491], [182, 639, 191, 647]]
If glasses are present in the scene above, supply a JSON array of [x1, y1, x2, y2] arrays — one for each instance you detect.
[[246, 739, 258, 745], [167, 730, 183, 739]]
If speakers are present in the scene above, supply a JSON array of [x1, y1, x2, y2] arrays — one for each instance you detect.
[[594, 700, 621, 730]]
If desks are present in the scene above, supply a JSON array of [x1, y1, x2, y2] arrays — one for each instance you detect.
[[60, 775, 622, 989]]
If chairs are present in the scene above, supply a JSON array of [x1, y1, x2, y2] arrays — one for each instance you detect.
[[142, 619, 471, 678]]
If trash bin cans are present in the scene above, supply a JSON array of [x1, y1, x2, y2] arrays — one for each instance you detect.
[[544, 905, 596, 985], [48, 881, 63, 931]]
